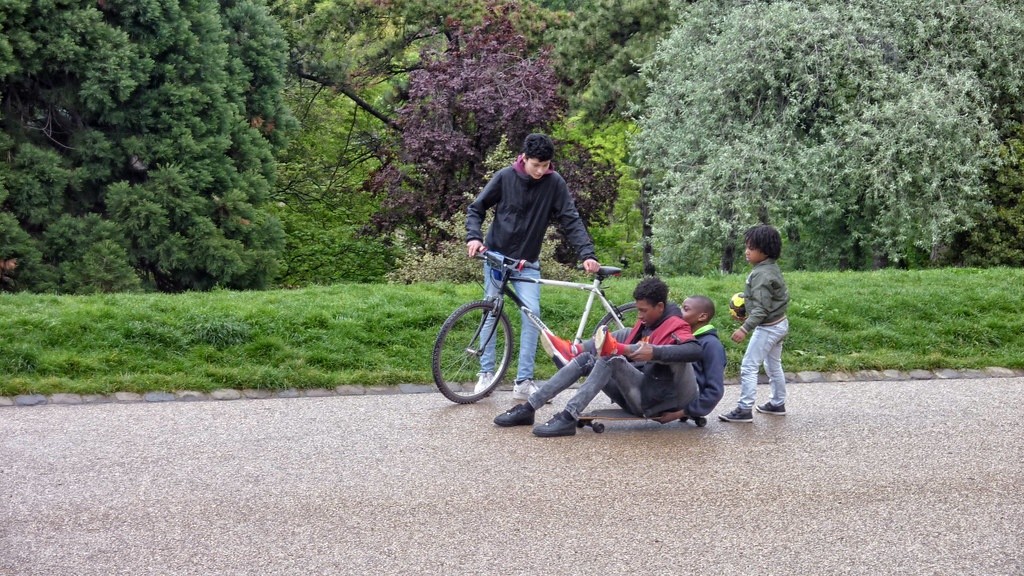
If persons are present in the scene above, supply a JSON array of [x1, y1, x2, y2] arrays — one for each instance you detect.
[[494, 276, 702, 437], [542, 295, 725, 424], [464, 133, 601, 403], [718, 226, 789, 422]]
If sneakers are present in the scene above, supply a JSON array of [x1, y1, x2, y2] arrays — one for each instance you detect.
[[718, 407, 754, 422], [594, 325, 617, 357], [532, 412, 576, 437], [539, 329, 575, 371], [512, 379, 554, 403], [474, 372, 494, 395], [494, 403, 535, 426], [756, 401, 787, 415]]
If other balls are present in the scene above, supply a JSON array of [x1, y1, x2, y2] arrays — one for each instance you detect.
[[729, 291, 746, 319]]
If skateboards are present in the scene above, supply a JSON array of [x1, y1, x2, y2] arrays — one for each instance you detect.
[[576, 409, 708, 433]]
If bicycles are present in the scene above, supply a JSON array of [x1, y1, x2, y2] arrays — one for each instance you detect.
[[432, 245, 637, 404]]
